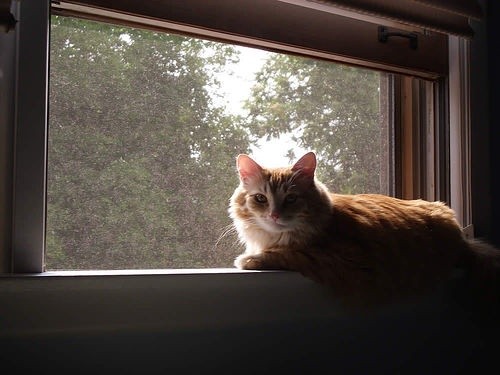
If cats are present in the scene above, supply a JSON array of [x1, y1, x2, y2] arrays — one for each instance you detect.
[[214, 153, 500, 282]]
